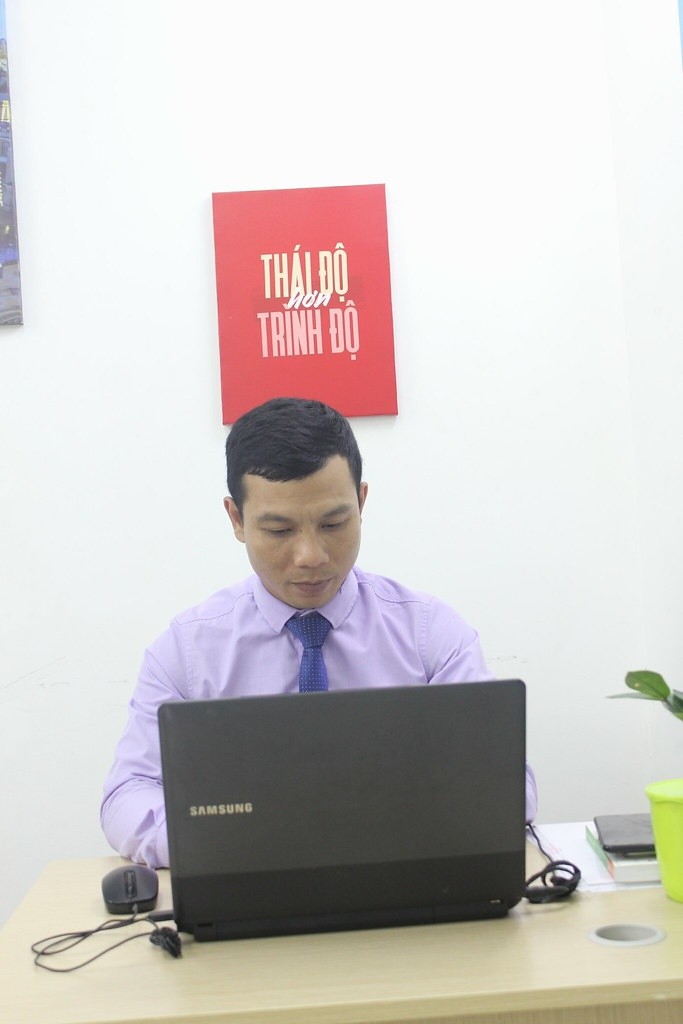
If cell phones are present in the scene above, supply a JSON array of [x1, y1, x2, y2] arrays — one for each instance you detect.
[[595, 812, 655, 852]]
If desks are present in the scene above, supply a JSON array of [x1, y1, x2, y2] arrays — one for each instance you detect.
[[0, 821, 683, 1024]]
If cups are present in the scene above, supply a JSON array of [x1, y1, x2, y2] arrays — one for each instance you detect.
[[645, 777, 683, 902]]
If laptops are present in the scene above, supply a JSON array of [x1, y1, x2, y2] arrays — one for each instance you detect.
[[156, 679, 527, 945]]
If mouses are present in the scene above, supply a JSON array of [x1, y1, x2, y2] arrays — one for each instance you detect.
[[101, 867, 159, 914]]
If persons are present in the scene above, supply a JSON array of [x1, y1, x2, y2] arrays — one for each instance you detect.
[[99, 398, 538, 872]]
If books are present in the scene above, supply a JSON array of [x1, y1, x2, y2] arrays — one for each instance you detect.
[[586, 821, 661, 882]]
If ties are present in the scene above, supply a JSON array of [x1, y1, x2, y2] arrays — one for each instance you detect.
[[286, 613, 332, 692]]
